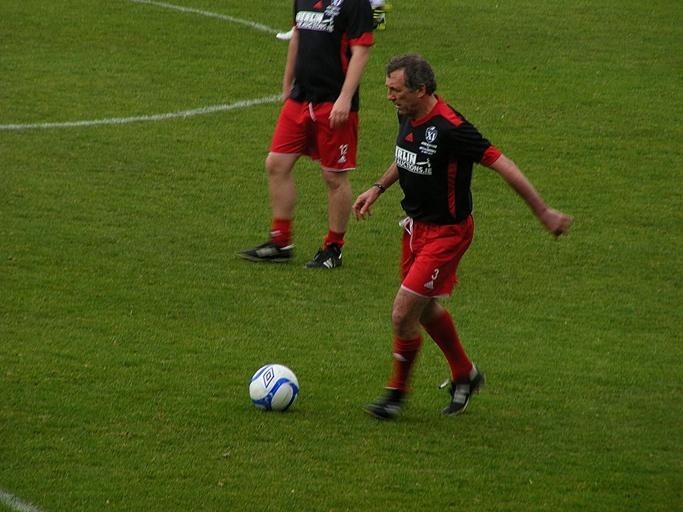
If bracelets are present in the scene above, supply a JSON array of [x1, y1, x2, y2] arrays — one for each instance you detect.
[[371, 182, 385, 193]]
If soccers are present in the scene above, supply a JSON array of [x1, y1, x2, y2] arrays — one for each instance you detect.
[[248, 363, 296, 410]]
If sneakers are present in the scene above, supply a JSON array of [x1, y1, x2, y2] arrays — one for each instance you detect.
[[364, 390, 399, 420], [441, 363, 481, 416], [370, 9, 385, 32], [274, 27, 294, 41], [302, 246, 342, 270], [235, 241, 297, 263]]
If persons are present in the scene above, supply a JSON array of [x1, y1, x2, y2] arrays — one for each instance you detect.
[[275, 0, 385, 41], [238, 0, 375, 269], [351, 53, 575, 423]]
